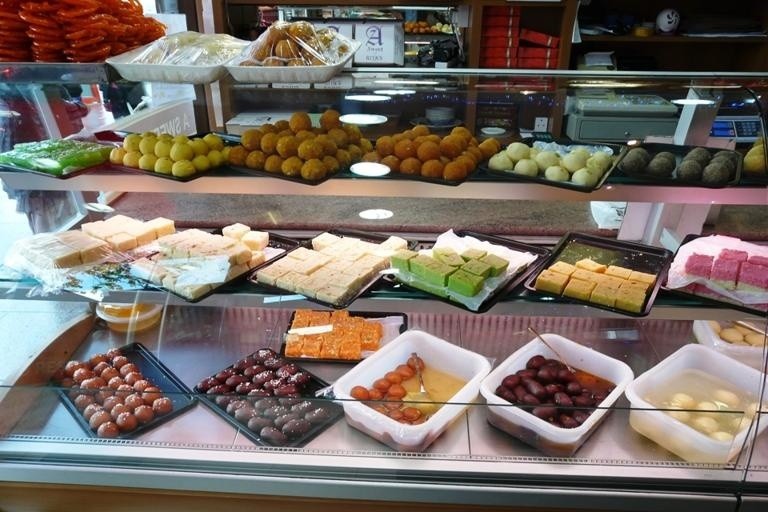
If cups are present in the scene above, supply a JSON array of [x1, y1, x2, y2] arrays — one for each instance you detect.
[[95, 302, 164, 333]]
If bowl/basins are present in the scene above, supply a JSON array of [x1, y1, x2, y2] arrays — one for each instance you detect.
[[426, 106, 456, 125]]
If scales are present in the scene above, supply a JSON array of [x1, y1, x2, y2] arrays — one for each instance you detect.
[[677, 105, 765, 142]]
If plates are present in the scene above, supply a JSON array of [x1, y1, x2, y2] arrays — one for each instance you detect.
[[411, 117, 461, 130], [480, 127, 505, 135]]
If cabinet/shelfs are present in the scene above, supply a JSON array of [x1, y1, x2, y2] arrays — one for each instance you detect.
[[210, 0, 482, 139], [550, 0, 767, 142], [1, 63, 768, 509]]
